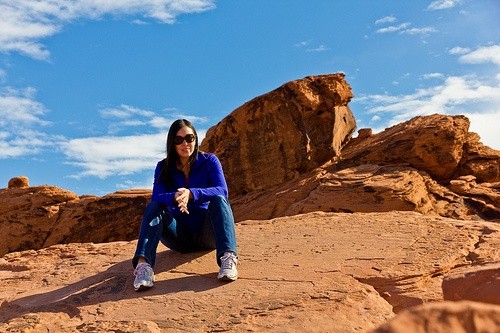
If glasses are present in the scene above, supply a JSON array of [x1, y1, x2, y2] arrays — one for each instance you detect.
[[173, 134, 195, 145]]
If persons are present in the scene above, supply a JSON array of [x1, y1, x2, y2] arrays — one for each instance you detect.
[[132, 119, 238, 292]]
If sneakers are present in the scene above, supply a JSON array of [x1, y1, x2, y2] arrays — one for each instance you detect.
[[133, 262, 155, 292], [217, 251, 239, 281]]
